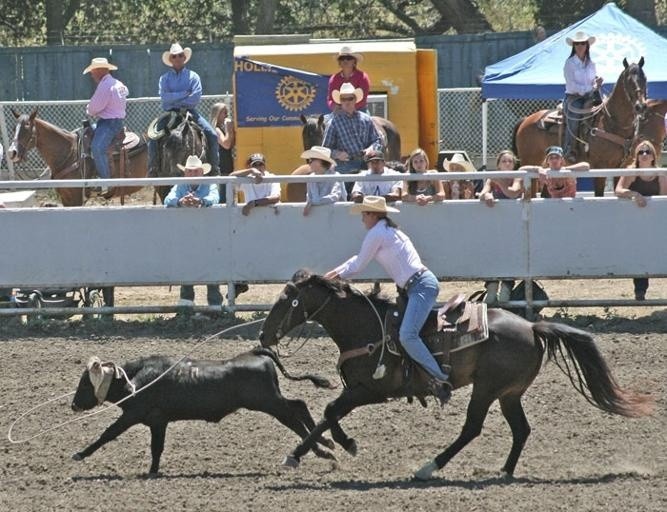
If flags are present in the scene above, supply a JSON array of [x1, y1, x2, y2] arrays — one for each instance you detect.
[[236, 55, 332, 127]]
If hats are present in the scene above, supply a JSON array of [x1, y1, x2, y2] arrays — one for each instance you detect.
[[333, 46, 364, 63], [443, 154, 476, 180], [162, 43, 192, 67], [364, 151, 383, 162], [566, 32, 596, 46], [82, 58, 118, 75], [350, 195, 400, 214], [331, 83, 364, 104], [544, 147, 563, 157], [249, 153, 265, 166], [176, 155, 211, 175], [300, 146, 338, 166]]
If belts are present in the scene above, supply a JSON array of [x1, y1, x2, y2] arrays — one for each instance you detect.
[[404, 268, 428, 291]]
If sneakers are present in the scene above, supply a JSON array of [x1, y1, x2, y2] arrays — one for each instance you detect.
[[438, 379, 451, 401], [212, 166, 221, 175], [146, 169, 159, 177]]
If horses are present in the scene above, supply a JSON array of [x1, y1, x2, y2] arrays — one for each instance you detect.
[[300, 112, 402, 162], [257, 266, 656, 481], [638, 98, 667, 168], [7, 104, 149, 206], [511, 56, 649, 197], [154, 119, 207, 205]]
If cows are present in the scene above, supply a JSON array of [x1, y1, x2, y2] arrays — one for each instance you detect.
[[70, 344, 341, 462]]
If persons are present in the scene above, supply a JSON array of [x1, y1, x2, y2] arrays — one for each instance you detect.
[[394, 148, 446, 205], [614, 139, 667, 301], [562, 30, 605, 157], [483, 149, 523, 305], [299, 143, 348, 217], [210, 100, 236, 202], [144, 42, 223, 178], [349, 142, 403, 301], [81, 56, 131, 196], [441, 152, 483, 200], [325, 46, 369, 111], [321, 82, 387, 172], [164, 154, 223, 321], [322, 195, 448, 385], [518, 144, 592, 200], [225, 153, 283, 303]]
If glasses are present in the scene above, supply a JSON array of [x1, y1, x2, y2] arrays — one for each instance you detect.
[[173, 54, 184, 58], [575, 42, 586, 46], [339, 55, 354, 60], [341, 97, 354, 101], [306, 159, 314, 164], [639, 150, 652, 155]]
